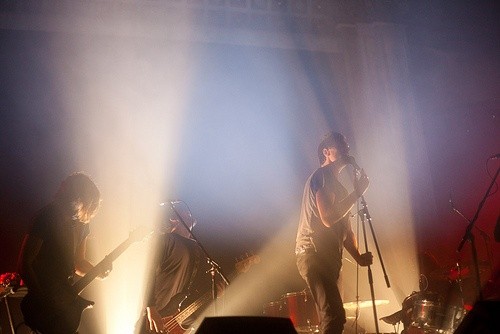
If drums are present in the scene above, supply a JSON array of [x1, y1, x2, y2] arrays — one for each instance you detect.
[[412, 293, 451, 330], [400, 292, 422, 333], [261, 291, 321, 332]]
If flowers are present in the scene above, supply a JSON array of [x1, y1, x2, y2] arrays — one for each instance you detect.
[[1, 272, 25, 291]]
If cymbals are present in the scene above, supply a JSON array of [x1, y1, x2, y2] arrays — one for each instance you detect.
[[344, 298, 389, 311]]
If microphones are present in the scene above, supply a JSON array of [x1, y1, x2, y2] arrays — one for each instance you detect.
[[346, 156, 360, 171], [448, 192, 453, 211], [160, 200, 183, 206]]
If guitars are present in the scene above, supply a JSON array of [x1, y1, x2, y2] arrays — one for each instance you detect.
[[21, 228, 148, 334], [145, 251, 261, 334]]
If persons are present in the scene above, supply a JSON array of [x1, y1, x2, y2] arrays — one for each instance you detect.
[[295, 130, 374, 334], [141, 199, 224, 334], [17, 173, 114, 334]]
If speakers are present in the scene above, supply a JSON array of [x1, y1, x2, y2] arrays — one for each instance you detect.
[[196, 316, 298, 334], [447, 299, 500, 334]]
